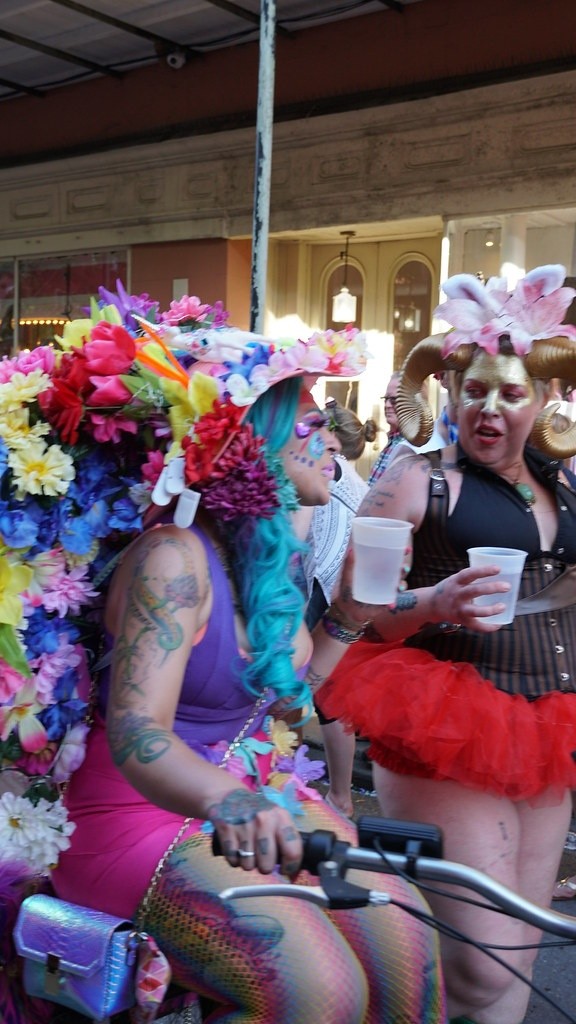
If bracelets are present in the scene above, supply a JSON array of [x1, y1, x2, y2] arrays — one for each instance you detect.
[[322, 612, 364, 645], [328, 602, 374, 631]]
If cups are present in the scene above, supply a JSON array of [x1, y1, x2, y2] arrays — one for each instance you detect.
[[351, 515, 415, 606], [467, 546, 529, 624]]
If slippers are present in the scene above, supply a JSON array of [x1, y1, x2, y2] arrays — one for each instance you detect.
[[552, 876, 576, 900]]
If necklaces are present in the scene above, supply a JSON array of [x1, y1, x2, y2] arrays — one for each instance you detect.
[[499, 465, 537, 508]]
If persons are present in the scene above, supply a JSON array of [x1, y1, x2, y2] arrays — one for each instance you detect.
[[38, 375, 451, 1024], [315, 349, 575, 1024], [288, 370, 576, 819]]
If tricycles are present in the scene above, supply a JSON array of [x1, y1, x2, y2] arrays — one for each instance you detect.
[[1, 816, 576, 1024]]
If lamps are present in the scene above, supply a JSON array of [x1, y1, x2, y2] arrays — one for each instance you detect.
[[331, 231, 357, 322]]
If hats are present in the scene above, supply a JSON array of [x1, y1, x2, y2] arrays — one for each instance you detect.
[[131, 331, 366, 541]]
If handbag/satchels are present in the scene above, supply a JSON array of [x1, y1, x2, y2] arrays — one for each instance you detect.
[[15, 894, 146, 1020]]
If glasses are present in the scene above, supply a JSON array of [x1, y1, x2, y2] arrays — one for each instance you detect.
[[380, 397, 398, 402]]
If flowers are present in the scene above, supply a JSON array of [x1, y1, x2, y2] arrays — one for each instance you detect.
[[0, 261, 576, 879]]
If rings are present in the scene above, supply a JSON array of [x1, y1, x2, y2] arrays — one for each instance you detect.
[[238, 848, 256, 857]]
